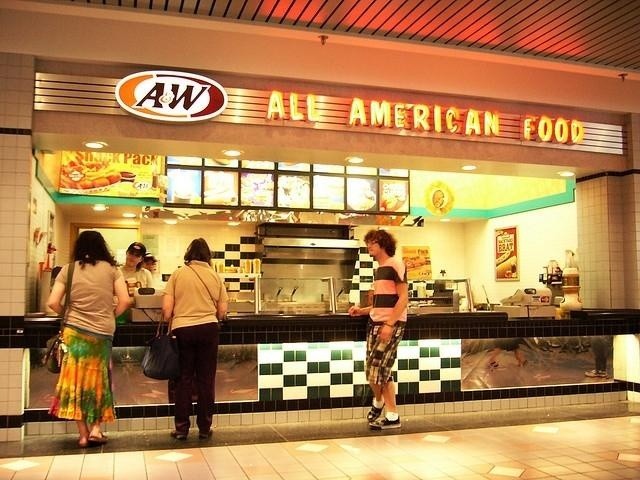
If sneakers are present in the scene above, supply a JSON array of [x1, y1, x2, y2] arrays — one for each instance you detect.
[[171, 430, 187, 440], [367, 402, 385, 422], [199, 430, 213, 439], [584, 369, 607, 377], [368, 416, 401, 430]]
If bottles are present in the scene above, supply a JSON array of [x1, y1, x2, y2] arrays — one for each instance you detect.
[[252, 259, 261, 273], [245, 260, 251, 274]]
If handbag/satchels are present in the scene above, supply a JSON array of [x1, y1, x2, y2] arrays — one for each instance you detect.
[[142, 313, 180, 380], [45, 334, 61, 374]]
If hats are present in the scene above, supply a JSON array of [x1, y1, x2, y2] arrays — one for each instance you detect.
[[127, 242, 146, 256], [144, 252, 153, 257]]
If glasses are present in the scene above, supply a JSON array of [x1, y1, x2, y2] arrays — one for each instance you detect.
[[365, 241, 379, 246]]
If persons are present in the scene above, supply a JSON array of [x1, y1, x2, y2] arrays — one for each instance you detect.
[[45, 230, 133, 450], [119, 241, 153, 308], [139, 250, 159, 277], [346, 227, 409, 429], [161, 238, 230, 442], [487, 337, 529, 373], [584, 336, 613, 380]]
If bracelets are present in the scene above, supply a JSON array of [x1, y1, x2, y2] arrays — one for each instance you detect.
[[383, 321, 395, 329]]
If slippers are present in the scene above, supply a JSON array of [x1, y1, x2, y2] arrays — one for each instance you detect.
[[77, 436, 89, 448], [87, 432, 108, 444]]
